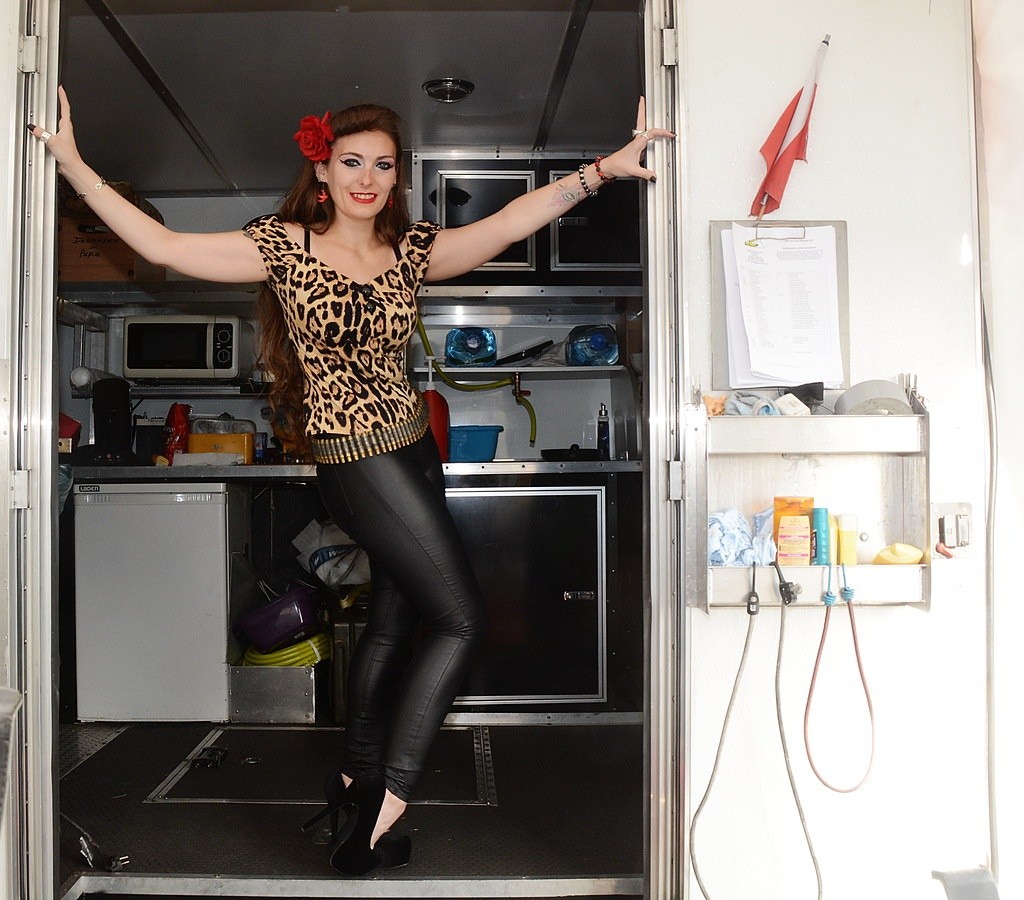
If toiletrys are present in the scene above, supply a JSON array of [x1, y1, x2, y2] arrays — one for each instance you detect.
[[775, 498, 924, 566]]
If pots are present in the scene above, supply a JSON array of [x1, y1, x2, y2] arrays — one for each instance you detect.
[[540, 443, 598, 461]]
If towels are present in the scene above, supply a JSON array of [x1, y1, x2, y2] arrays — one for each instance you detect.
[[703, 391, 781, 416], [708, 506, 779, 567]]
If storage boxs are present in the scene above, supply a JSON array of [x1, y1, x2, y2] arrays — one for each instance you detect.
[[227, 656, 316, 725]]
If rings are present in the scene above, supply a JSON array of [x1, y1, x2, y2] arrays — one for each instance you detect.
[[645, 131, 650, 140], [632, 130, 642, 137], [40, 131, 51, 143]]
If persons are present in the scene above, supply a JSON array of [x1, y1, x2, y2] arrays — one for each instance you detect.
[[27, 85, 677, 876]]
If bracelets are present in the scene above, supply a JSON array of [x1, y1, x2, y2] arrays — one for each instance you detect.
[[78, 178, 106, 200], [595, 155, 617, 182], [579, 164, 598, 196]]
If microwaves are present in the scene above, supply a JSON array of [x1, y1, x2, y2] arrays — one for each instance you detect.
[[121, 314, 242, 383]]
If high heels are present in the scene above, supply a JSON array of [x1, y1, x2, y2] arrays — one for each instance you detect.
[[297, 763, 386, 879], [319, 767, 414, 871]]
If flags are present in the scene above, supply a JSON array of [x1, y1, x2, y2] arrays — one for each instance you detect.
[[750, 74, 818, 217]]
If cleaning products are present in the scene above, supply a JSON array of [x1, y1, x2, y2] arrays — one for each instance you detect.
[[421, 355, 451, 461]]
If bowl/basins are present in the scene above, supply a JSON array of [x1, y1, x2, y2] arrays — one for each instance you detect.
[[448, 425, 504, 462]]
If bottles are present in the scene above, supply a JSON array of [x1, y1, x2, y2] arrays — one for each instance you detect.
[[597, 403, 611, 459], [444, 327, 498, 367], [614, 403, 628, 460], [564, 325, 618, 365], [812, 508, 858, 566]]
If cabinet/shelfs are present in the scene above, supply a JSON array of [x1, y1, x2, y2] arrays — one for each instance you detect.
[[61, 277, 284, 403], [408, 148, 644, 290], [702, 411, 923, 613], [403, 482, 614, 712]]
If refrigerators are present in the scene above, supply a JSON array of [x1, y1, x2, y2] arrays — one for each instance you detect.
[[72, 482, 230, 726]]
